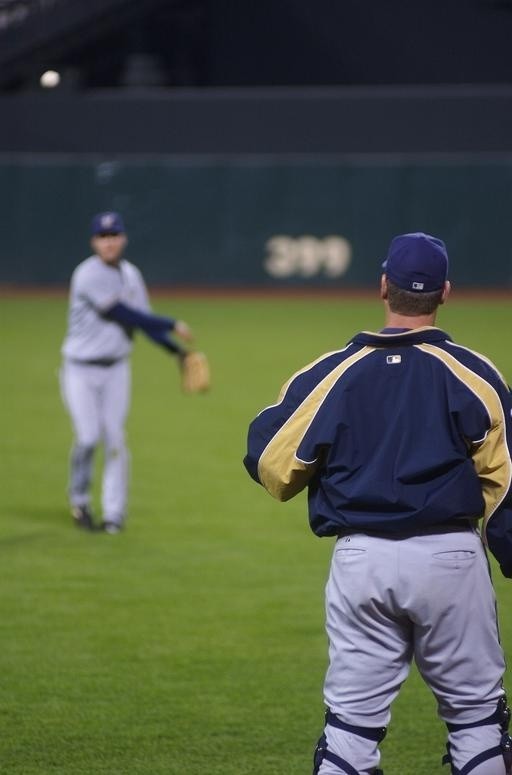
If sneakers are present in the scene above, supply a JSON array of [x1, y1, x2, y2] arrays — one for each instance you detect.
[[71, 503, 94, 532], [103, 521, 120, 534]]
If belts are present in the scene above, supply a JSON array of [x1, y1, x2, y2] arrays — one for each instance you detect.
[[75, 358, 122, 368]]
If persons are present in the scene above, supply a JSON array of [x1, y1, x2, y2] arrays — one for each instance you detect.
[[58, 211, 213, 535], [242, 229, 512, 774]]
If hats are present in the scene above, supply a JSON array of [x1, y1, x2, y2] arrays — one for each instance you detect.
[[383, 232, 449, 290], [89, 211, 126, 237]]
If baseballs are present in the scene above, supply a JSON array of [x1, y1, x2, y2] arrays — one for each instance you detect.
[[41, 71, 59, 89]]
